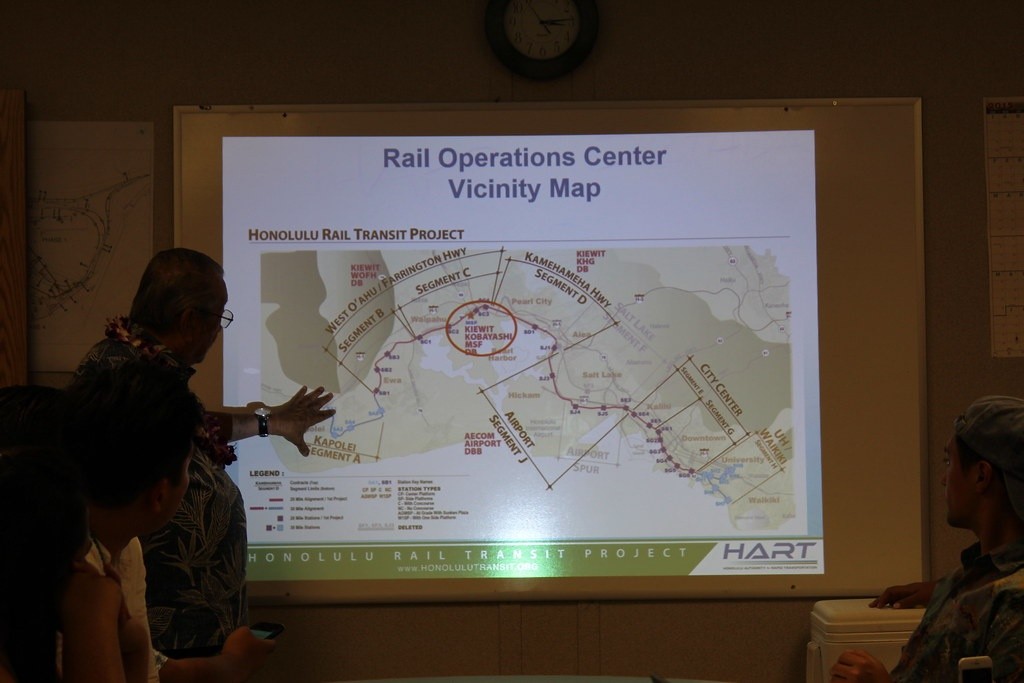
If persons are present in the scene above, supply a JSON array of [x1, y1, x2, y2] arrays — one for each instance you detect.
[[826, 394, 1024, 683], [73, 247, 337, 660], [0, 366, 278, 682]]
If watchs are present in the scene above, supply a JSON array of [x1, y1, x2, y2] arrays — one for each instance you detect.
[[255, 405, 274, 438]]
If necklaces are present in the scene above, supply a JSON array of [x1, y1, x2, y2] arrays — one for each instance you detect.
[[102, 315, 238, 467]]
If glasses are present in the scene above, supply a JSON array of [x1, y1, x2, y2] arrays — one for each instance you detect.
[[176, 306, 233, 328]]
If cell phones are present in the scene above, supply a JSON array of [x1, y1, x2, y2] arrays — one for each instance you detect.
[[958, 656, 993, 683], [249, 621, 286, 638]]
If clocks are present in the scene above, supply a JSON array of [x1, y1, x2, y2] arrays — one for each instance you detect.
[[483, 0, 599, 79]]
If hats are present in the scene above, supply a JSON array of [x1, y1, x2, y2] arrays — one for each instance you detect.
[[953, 395, 1024, 520]]
